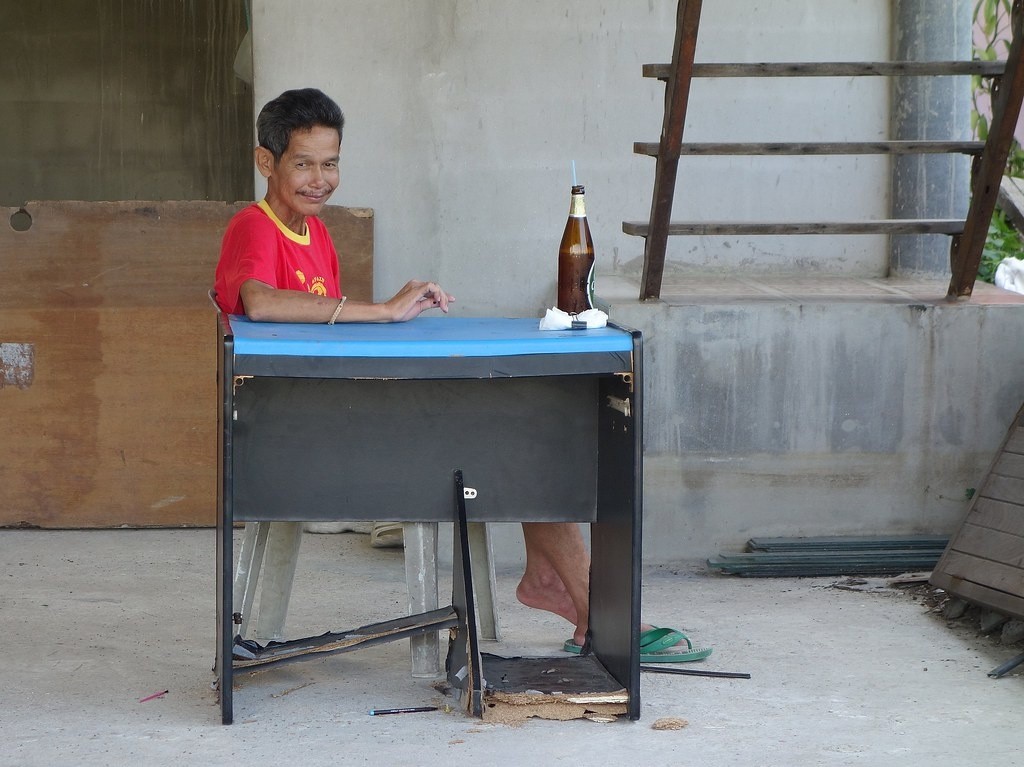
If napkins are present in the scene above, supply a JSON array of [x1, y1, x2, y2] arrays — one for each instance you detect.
[[538, 307, 608, 331]]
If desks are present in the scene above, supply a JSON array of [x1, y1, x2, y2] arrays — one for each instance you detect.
[[214, 316, 642, 726]]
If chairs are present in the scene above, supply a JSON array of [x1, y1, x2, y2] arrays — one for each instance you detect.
[[208, 287, 497, 678]]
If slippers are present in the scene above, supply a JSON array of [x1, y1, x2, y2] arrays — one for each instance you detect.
[[564, 624, 660, 653], [641, 628, 713, 662]]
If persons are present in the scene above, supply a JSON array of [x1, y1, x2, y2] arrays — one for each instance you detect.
[[215, 89, 712, 662]]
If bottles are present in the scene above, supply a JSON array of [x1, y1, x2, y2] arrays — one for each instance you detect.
[[558, 185, 595, 315]]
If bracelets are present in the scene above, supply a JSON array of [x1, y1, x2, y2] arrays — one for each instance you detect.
[[328, 296, 347, 325]]
